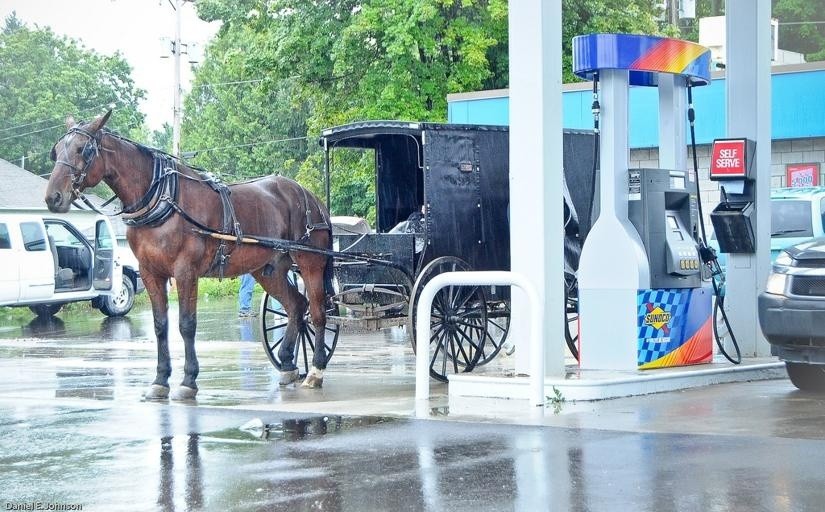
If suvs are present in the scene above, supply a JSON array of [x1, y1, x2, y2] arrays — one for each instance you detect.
[[705, 183, 825, 296], [752, 233, 825, 391]]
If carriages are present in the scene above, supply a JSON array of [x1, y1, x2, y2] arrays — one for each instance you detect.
[[40, 106, 601, 404]]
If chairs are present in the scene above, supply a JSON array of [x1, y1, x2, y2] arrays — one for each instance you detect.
[[48, 235, 74, 289]]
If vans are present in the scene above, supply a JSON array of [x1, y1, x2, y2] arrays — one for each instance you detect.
[[0, 205, 147, 323]]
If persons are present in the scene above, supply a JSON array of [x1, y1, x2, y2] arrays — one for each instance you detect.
[[270, 297, 285, 320], [238, 272, 259, 318]]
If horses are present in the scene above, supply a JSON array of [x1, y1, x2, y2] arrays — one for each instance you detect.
[[42, 107, 335, 403]]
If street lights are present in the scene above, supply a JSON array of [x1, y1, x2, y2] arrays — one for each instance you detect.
[[153, 9, 198, 157]]
